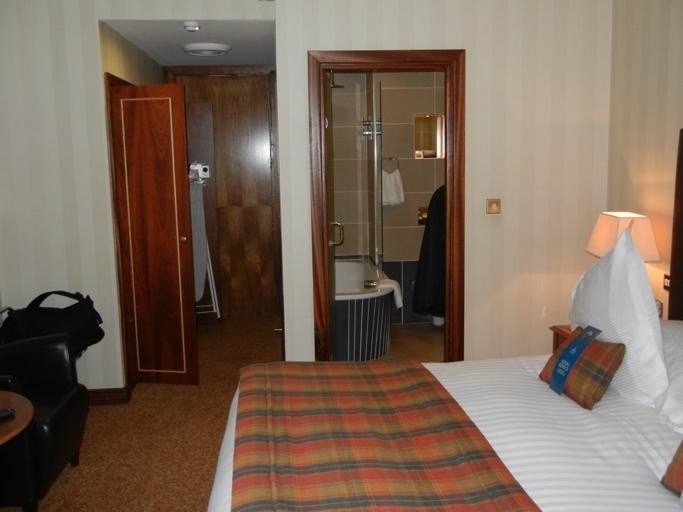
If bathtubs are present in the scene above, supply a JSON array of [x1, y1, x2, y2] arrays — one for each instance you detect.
[[335, 258, 394, 364]]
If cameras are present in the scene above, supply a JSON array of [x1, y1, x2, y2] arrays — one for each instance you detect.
[[190, 162, 210, 179]]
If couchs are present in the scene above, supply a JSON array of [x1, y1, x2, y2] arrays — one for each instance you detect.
[[0, 334, 89, 508]]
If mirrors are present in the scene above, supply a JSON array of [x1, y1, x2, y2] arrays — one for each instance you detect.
[[412, 113, 446, 160]]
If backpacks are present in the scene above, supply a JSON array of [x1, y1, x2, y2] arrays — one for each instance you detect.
[[0, 290, 105, 367]]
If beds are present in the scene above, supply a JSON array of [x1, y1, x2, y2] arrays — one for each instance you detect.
[[206, 132, 683, 512]]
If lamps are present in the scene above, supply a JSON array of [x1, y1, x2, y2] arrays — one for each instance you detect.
[[184, 43, 231, 57], [184, 21, 199, 32]]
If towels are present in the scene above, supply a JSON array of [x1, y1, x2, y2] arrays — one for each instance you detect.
[[369, 279, 404, 309], [382, 169, 405, 207]]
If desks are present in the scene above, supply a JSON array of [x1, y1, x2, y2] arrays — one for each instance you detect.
[[0, 390, 38, 512]]
